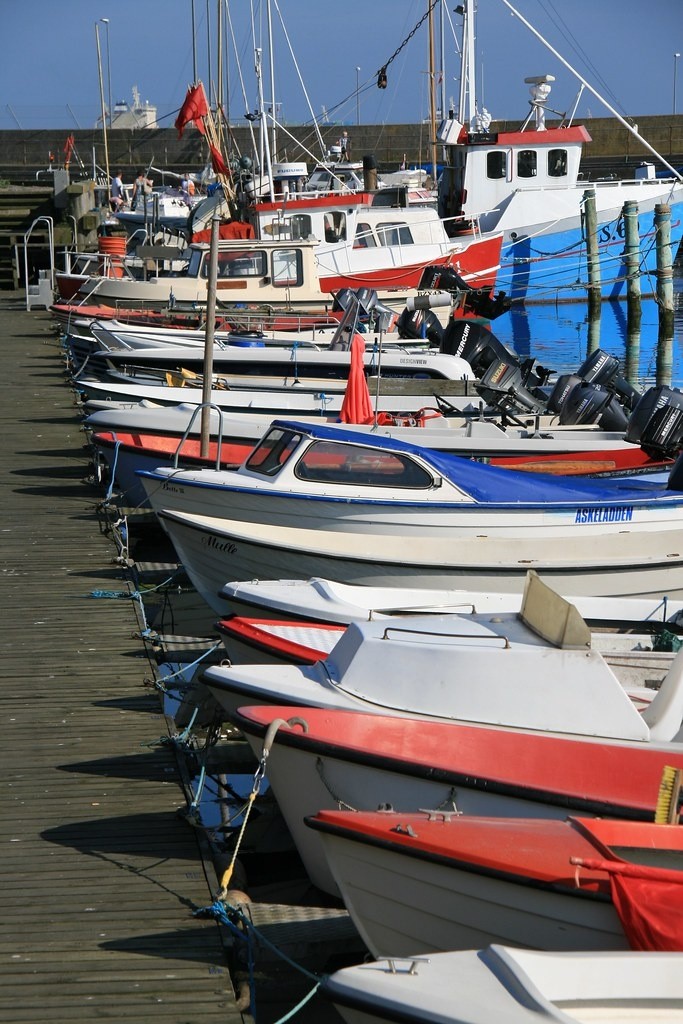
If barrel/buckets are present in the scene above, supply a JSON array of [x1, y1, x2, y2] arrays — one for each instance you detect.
[[98, 236, 128, 278]]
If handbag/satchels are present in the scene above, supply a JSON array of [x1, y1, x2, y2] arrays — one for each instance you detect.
[[189, 181, 194, 195], [143, 179, 152, 194]]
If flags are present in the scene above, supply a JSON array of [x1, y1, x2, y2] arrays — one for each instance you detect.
[[174, 83, 210, 139]]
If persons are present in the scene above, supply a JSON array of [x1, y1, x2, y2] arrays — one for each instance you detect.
[[179, 173, 194, 211], [108, 169, 129, 213], [336, 131, 351, 163], [130, 171, 153, 211]]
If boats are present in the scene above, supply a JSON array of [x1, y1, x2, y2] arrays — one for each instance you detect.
[[230, 706, 683, 902], [304, 808, 683, 961], [318, 943, 683, 1024], [195, 568, 683, 755], [48, 1, 683, 510], [213, 576, 683, 690], [156, 507, 683, 617], [135, 403, 683, 537]]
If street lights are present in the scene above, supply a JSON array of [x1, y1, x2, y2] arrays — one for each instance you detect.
[[356, 67, 360, 126], [674, 54, 681, 114], [101, 19, 112, 129]]
